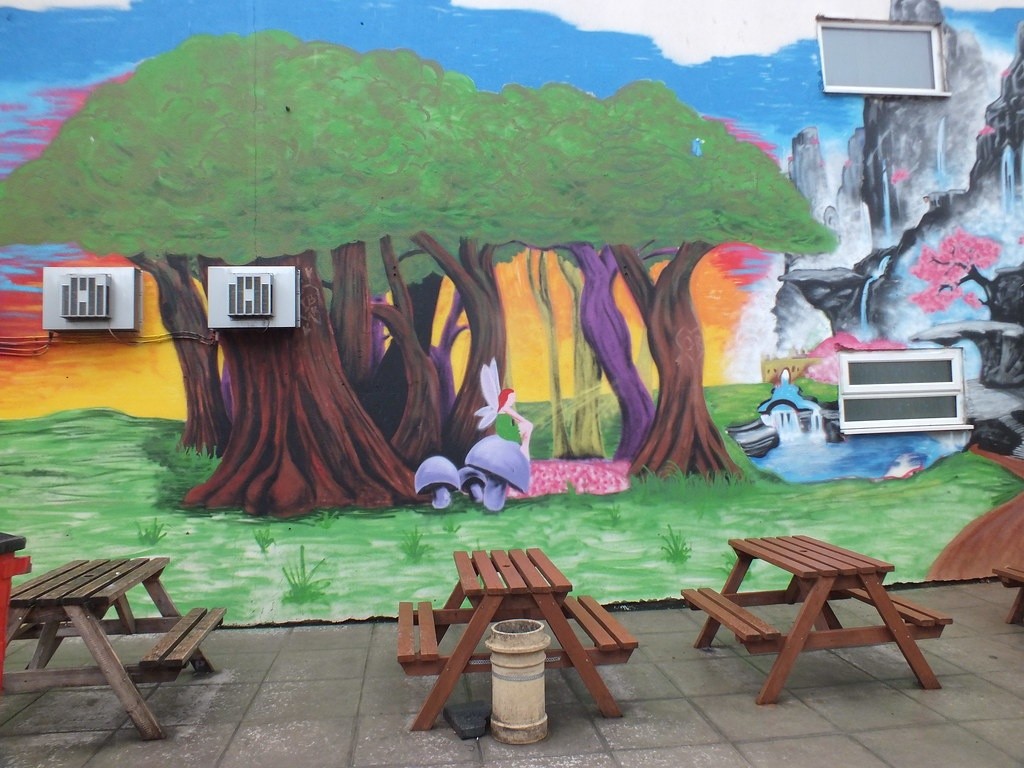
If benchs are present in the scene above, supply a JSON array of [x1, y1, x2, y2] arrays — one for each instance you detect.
[[682, 588, 783, 642], [846, 589, 954, 626], [563, 595, 638, 651], [140, 607, 226, 670], [397, 601, 439, 662]]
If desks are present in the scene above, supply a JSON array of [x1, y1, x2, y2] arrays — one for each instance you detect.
[[0, 557, 222, 740], [688, 536, 945, 706], [399, 547, 635, 733]]
